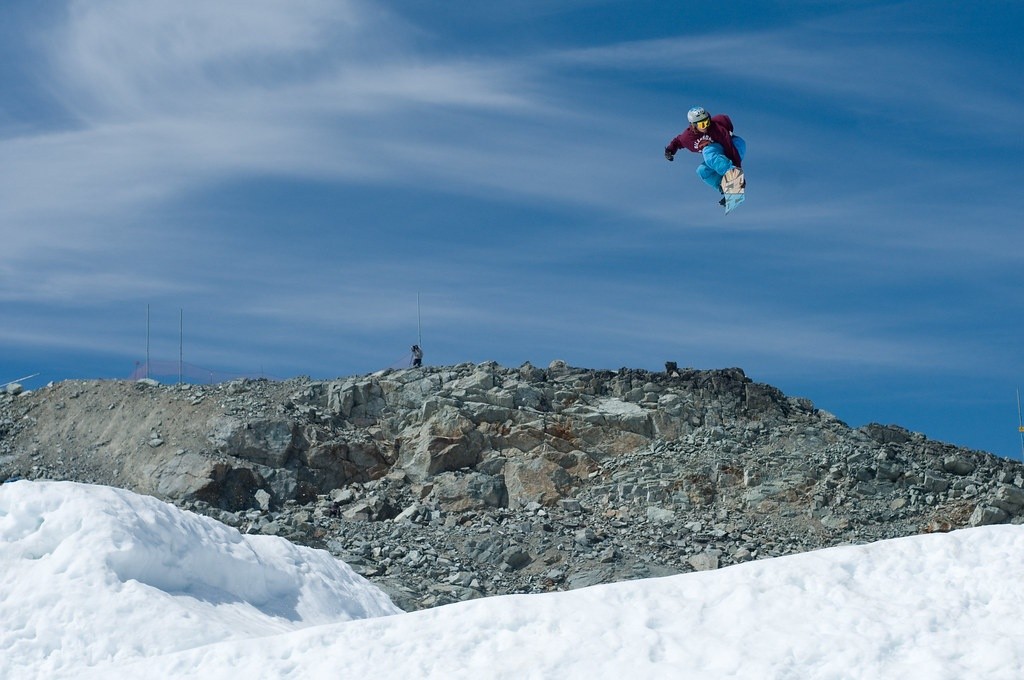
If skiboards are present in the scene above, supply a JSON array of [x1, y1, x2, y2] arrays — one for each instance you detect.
[[406, 364, 424, 371]]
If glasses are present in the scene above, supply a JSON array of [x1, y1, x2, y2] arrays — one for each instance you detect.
[[697, 118, 710, 129]]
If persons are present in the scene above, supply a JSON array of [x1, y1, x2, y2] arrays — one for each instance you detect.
[[411, 346, 423, 367], [666, 106, 745, 206]]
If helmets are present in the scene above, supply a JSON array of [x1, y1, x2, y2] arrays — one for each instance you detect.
[[687, 106, 709, 123]]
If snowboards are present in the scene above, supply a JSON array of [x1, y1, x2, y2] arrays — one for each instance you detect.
[[721, 167, 745, 216]]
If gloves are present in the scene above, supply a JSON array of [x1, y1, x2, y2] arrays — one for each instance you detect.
[[664, 147, 674, 161]]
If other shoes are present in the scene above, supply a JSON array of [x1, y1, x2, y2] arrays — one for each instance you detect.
[[719, 196, 726, 205], [741, 179, 746, 188]]
